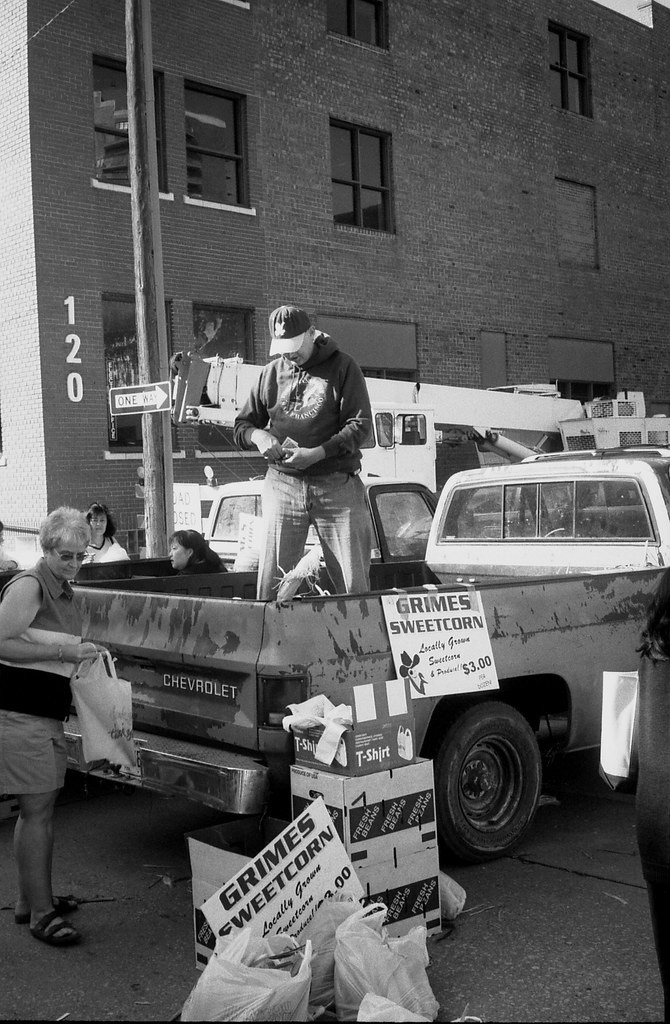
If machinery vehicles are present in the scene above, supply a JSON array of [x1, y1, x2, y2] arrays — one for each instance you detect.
[[135, 351, 670, 559]]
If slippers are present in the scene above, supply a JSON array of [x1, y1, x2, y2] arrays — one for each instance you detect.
[[14, 895, 80, 925], [31, 910, 82, 945]]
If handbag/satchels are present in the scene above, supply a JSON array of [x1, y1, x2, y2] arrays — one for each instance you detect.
[[597, 671, 639, 792], [72, 650, 137, 768], [180, 893, 438, 1022]]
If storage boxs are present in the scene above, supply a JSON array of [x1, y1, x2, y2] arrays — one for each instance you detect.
[[355, 845, 442, 938], [290, 757, 437, 869], [292, 677, 416, 777], [184, 818, 290, 973]]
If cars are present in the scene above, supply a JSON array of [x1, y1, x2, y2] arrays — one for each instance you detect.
[[396, 444, 670, 557]]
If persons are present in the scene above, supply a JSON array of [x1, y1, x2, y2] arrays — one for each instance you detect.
[[234, 305, 373, 599], [0, 507, 108, 945], [0, 521, 18, 570], [81, 503, 121, 564], [169, 530, 228, 575], [635, 566, 670, 1022]]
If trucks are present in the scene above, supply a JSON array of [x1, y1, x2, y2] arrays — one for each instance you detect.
[[0, 480, 439, 594], [63, 456, 670, 867]]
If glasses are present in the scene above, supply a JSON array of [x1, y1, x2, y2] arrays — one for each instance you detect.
[[52, 548, 89, 562]]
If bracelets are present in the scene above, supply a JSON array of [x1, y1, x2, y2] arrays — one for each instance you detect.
[[58, 645, 62, 659], [12, 561, 18, 568]]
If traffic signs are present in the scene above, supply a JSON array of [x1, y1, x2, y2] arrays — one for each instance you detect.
[[109, 382, 172, 415]]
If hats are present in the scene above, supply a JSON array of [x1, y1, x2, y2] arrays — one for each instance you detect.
[[269, 305, 310, 356]]
